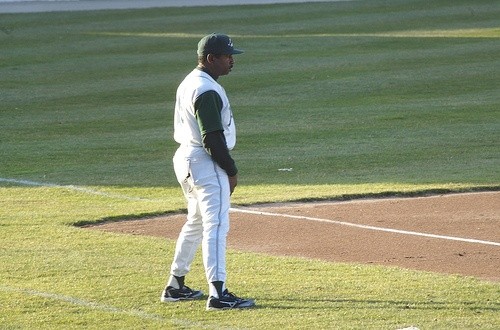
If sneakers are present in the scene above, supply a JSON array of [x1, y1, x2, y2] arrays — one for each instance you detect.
[[206, 289, 254, 311], [161, 285, 203, 302]]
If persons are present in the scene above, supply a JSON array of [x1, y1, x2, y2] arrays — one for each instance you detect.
[[161, 32, 256, 310]]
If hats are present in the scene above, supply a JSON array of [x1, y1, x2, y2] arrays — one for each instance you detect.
[[197, 32, 244, 56]]
